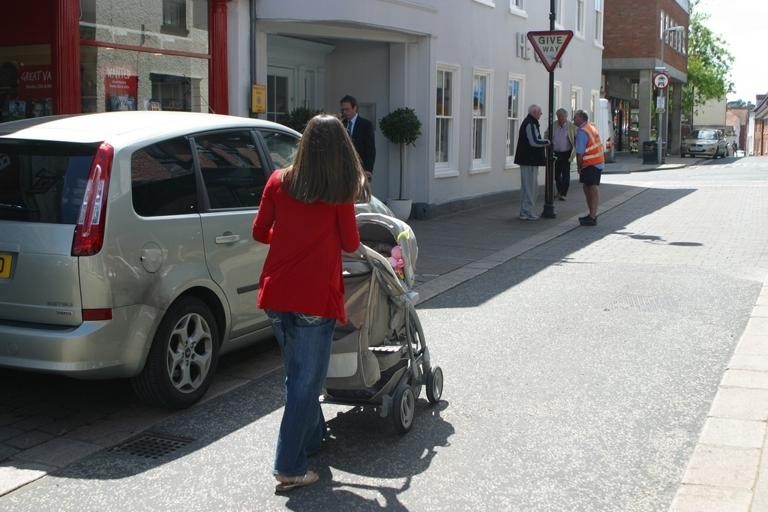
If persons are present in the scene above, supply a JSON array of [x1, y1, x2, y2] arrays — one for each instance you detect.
[[30, 101, 47, 116], [731, 140, 737, 156], [250, 115, 368, 491], [544, 108, 575, 199], [12, 102, 24, 115], [572, 109, 605, 225], [514, 105, 550, 219], [338, 95, 375, 181]]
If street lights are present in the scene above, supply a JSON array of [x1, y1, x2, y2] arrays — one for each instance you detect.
[[655, 25, 685, 164]]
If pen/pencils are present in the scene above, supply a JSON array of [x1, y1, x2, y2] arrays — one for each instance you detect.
[[642, 141, 666, 163]]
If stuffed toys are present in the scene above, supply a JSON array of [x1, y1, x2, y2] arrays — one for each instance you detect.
[[386, 247, 403, 277]]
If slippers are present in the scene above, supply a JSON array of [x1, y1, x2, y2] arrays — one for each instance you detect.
[[276, 473, 319, 491]]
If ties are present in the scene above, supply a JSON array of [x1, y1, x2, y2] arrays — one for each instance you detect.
[[347, 121, 352, 135]]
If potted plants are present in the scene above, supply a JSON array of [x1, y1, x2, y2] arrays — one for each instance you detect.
[[377, 107, 423, 223]]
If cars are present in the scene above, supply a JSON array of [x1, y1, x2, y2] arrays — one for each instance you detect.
[[680, 127, 728, 161]]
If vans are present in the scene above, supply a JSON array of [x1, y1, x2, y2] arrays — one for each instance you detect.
[[1, 109, 397, 408], [599, 97, 615, 163]]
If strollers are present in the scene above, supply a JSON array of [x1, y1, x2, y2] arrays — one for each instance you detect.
[[321, 211, 444, 437]]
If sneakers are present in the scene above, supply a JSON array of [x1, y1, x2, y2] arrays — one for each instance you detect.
[[520, 214, 537, 221], [579, 215, 596, 225]]
